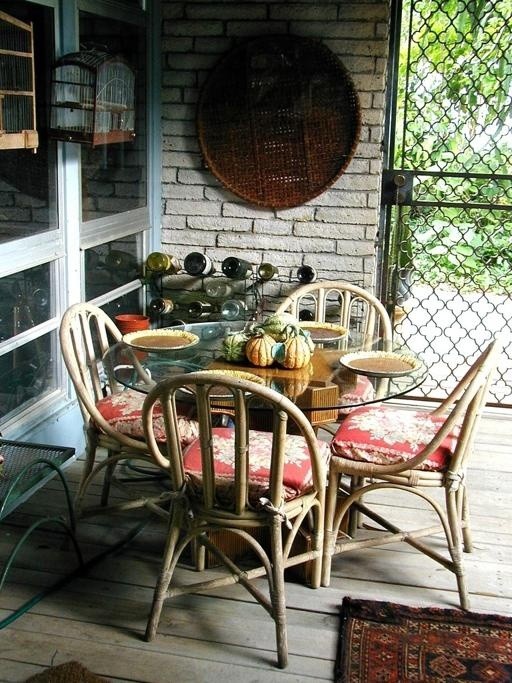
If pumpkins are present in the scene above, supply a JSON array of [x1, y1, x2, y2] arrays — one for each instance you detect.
[[222, 317, 314, 369], [247, 362, 313, 403]]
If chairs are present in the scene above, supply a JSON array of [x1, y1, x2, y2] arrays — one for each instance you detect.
[[321, 339, 502, 611], [274, 281, 393, 529], [59, 302, 222, 550], [143, 373, 333, 669]]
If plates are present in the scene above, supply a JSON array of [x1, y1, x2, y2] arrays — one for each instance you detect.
[[295, 320, 348, 342], [339, 350, 422, 377], [123, 329, 201, 350]]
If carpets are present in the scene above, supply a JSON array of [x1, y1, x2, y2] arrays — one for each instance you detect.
[[333, 596, 512, 683]]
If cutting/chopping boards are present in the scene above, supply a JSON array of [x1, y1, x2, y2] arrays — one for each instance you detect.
[[204, 343, 352, 386]]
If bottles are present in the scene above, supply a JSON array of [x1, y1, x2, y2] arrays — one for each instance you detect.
[[146, 252, 319, 321]]
